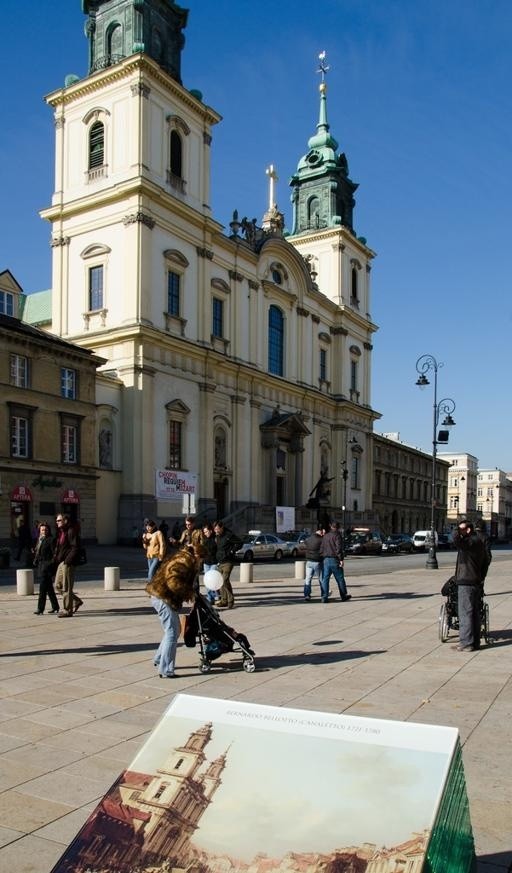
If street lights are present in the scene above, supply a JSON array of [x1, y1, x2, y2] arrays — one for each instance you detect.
[[339, 458, 350, 545], [413, 353, 458, 567]]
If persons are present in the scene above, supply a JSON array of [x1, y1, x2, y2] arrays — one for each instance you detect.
[[140, 517, 243, 677], [474, 519, 491, 572], [304, 526, 332, 601], [451, 520, 488, 652], [14, 513, 83, 618], [319, 521, 352, 603]]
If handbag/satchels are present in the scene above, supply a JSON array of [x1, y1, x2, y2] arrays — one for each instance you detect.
[[71, 546, 88, 566], [177, 599, 206, 650]]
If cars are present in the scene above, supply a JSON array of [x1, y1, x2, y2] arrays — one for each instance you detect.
[[231, 527, 417, 561], [438, 533, 454, 548]]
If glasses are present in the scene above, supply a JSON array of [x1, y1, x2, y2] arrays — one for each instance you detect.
[[55, 518, 65, 522]]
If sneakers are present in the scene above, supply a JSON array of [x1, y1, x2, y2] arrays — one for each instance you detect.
[[58, 609, 74, 618], [73, 600, 84, 613], [322, 598, 331, 603], [454, 643, 481, 652], [304, 594, 312, 601], [340, 593, 352, 602], [328, 589, 333, 596], [208, 595, 234, 610]]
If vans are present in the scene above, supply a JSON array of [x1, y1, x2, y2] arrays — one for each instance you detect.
[[412, 530, 440, 549]]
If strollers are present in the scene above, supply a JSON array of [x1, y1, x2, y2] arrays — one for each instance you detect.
[[178, 587, 259, 674]]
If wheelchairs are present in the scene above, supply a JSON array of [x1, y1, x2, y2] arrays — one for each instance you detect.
[[436, 573, 496, 646]]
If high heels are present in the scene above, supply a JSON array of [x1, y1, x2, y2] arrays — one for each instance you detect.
[[153, 662, 159, 668], [33, 608, 44, 615], [158, 671, 182, 679], [48, 606, 59, 614]]
